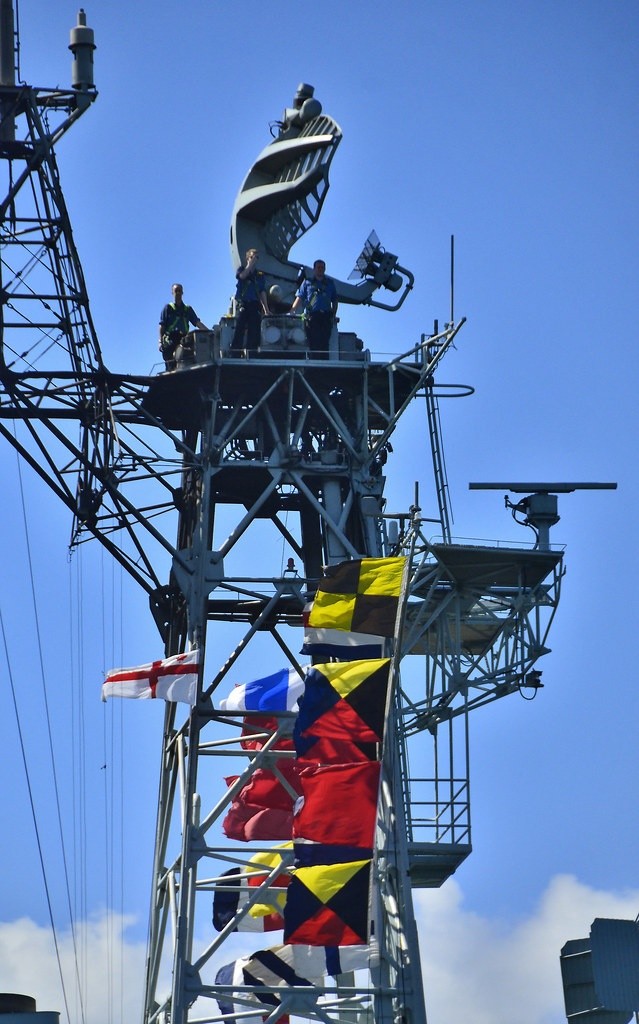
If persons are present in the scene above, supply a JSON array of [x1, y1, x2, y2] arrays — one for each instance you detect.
[[227, 248, 273, 358], [290, 259, 338, 360], [158, 283, 209, 372]]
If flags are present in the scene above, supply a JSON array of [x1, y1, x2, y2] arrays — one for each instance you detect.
[[225, 662, 312, 713], [215, 944, 370, 1024], [308, 557, 406, 638], [101, 649, 200, 707], [212, 840, 375, 947], [224, 658, 393, 848]]
